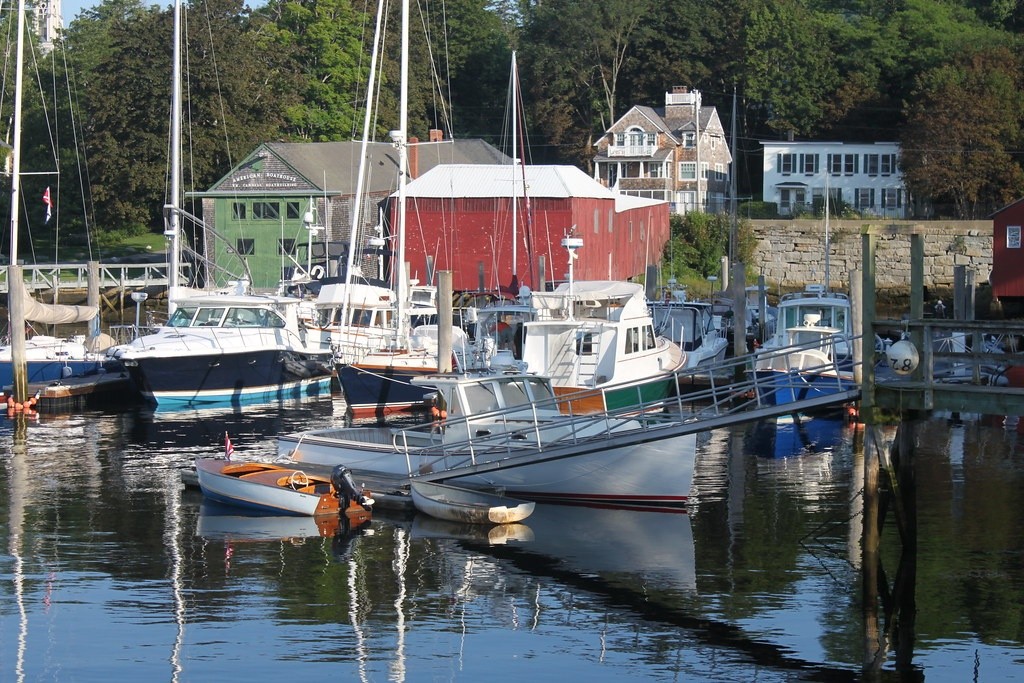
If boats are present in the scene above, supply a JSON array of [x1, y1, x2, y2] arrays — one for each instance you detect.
[[196, 504, 338, 543], [195, 453, 364, 515], [409, 480, 537, 525], [392, 498, 700, 599], [411, 512, 535, 546]]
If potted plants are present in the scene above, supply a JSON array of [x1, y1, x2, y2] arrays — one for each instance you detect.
[[948, 234, 970, 266]]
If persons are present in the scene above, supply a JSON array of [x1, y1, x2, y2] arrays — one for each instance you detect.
[[932, 300, 948, 338], [513, 322, 526, 361], [490, 320, 516, 356]]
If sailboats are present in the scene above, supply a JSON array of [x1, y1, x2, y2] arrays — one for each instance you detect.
[[0, 0, 903, 503]]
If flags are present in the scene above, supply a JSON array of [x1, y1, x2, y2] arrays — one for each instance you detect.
[[225, 433, 234, 461], [43, 187, 54, 225]]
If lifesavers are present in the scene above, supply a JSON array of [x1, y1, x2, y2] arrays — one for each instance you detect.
[[311, 265, 325, 279], [490, 321, 511, 333]]
